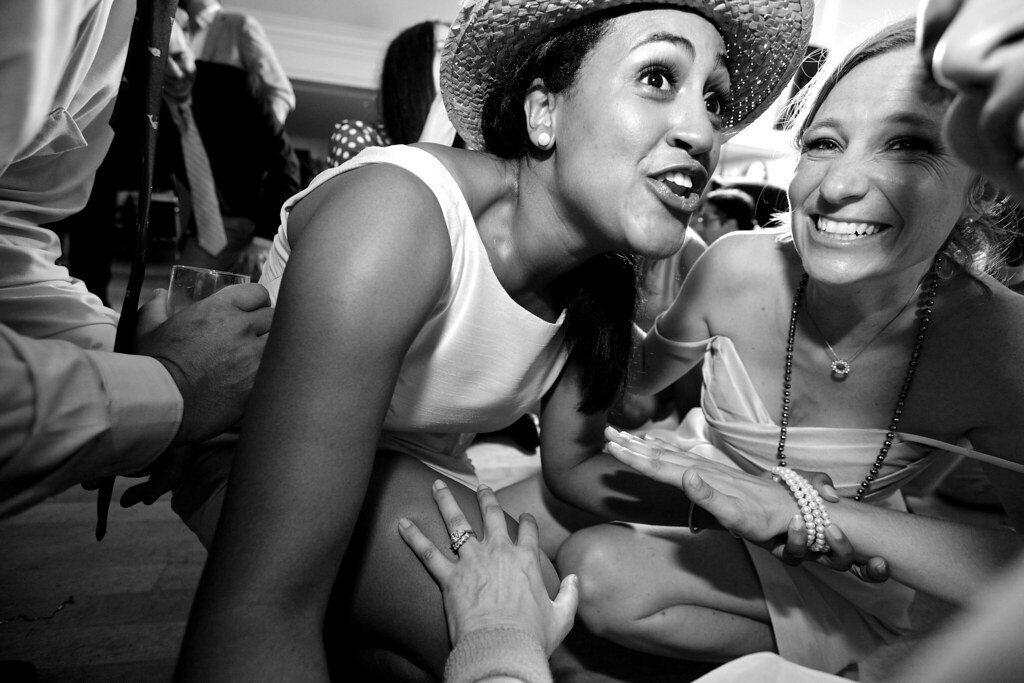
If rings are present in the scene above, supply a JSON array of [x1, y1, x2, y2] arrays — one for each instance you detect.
[[449, 528, 477, 552]]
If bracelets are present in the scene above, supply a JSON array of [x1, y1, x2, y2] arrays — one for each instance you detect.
[[688, 499, 712, 535], [770, 466, 831, 553]]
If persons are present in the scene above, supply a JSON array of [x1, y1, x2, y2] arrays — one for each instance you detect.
[[606, 223, 712, 430], [692, 188, 762, 247], [170, 2, 893, 683], [397, 1, 1023, 682], [38, 0, 153, 311], [480, 25, 1024, 683], [175, 0, 302, 126], [0, 0, 277, 683], [323, 20, 457, 167], [134, 18, 301, 319]]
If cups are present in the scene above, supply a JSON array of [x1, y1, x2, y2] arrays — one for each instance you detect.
[[164, 264, 251, 319]]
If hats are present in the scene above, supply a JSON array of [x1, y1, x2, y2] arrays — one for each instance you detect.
[[440, 1, 815, 150]]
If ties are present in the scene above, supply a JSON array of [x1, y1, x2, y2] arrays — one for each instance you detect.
[[94, 0, 176, 541], [171, 101, 228, 258]]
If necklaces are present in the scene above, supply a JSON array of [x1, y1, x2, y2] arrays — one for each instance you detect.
[[777, 257, 944, 502], [803, 274, 929, 375]]
